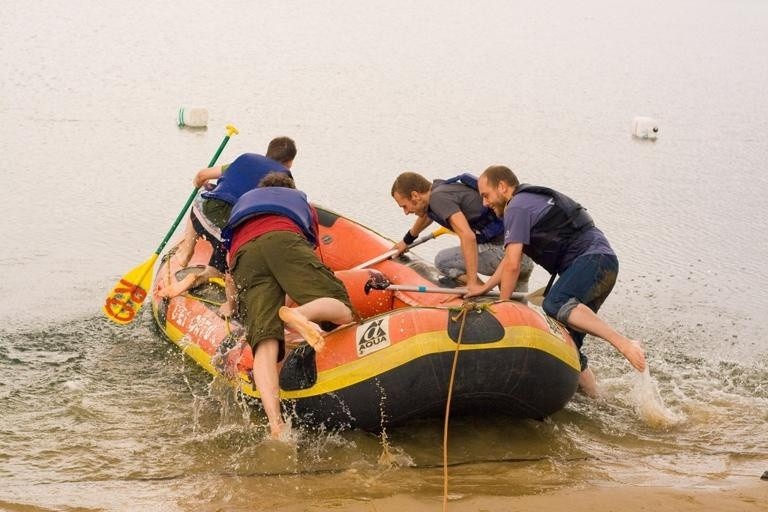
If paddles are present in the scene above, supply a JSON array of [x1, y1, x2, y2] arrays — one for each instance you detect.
[[104, 126, 240, 325], [384, 285, 547, 306], [350, 227, 459, 270]]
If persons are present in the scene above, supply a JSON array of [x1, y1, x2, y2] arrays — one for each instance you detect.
[[152, 137, 298, 299], [390, 171, 535, 309], [219, 169, 356, 448], [455, 165, 646, 400]]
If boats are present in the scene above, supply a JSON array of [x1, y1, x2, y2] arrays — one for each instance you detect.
[[141, 192, 586, 442]]
